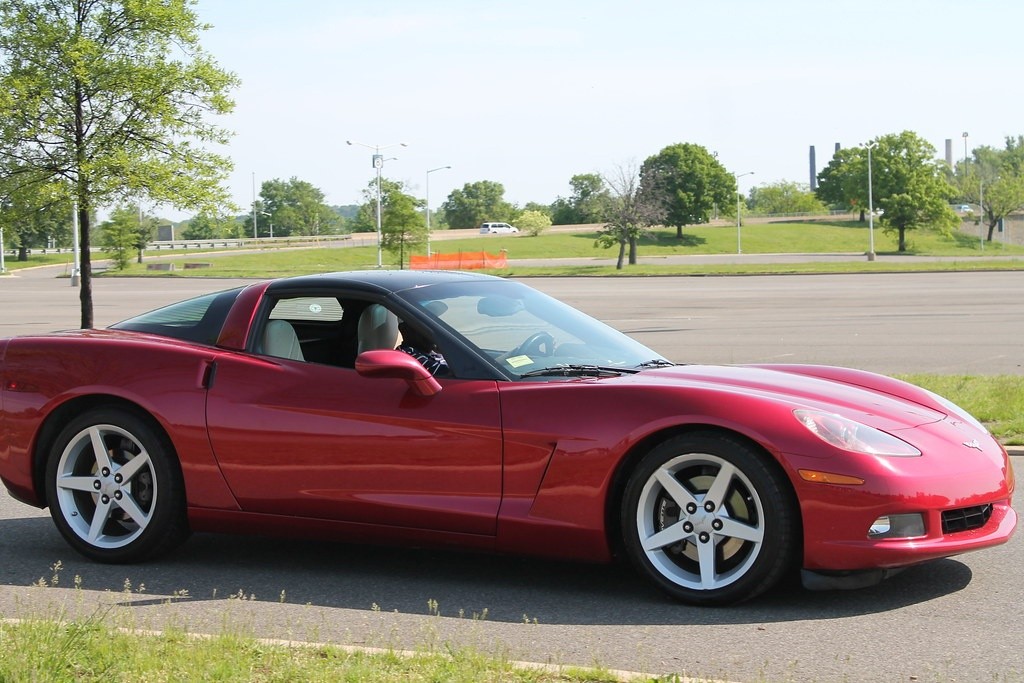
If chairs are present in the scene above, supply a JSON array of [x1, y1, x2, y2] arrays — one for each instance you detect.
[[359, 304, 399, 357], [261, 320, 307, 361]]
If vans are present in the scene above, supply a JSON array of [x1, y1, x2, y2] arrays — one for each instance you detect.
[[480, 222, 519, 234]]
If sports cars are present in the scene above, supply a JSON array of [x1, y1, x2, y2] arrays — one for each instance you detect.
[[0, 269, 1020, 611]]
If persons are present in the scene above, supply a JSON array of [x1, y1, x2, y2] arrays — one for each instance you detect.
[[395, 301, 452, 379]]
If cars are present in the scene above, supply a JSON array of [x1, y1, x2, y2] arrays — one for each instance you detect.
[[955, 204, 973, 215]]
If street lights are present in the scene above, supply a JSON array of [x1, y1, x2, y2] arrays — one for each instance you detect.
[[736, 172, 756, 254], [962, 131, 969, 177], [346, 140, 408, 268], [859, 142, 879, 261], [426, 165, 453, 256]]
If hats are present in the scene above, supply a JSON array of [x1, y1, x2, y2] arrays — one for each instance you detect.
[[398, 300, 447, 324]]
